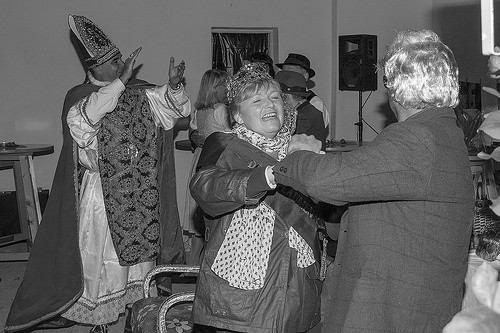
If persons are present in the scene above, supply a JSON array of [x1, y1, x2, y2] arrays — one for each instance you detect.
[[182, 53, 331, 333], [3, 13, 191, 333], [271, 30, 476, 333]]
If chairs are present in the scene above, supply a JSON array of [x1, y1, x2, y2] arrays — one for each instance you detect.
[[124, 265, 201, 333]]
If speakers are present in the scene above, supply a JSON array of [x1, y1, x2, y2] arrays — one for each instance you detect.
[[338, 34, 378, 91]]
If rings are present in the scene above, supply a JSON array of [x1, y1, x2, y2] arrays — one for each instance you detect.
[[128, 53, 133, 58]]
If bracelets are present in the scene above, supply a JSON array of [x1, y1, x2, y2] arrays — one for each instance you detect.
[[168, 81, 180, 90]]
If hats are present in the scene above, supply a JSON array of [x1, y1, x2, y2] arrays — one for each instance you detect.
[[275, 53, 315, 79], [274, 71, 311, 98], [68, 14, 121, 70]]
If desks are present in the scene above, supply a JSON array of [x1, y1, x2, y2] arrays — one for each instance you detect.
[[0, 143, 55, 261]]
